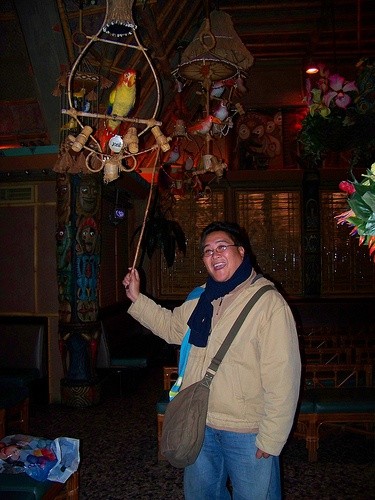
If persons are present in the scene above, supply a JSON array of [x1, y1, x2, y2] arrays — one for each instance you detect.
[[122, 219, 302, 500]]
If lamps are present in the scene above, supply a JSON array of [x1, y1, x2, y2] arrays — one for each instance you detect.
[[305, 61, 319, 74]]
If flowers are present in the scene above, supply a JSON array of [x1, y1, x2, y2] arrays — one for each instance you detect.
[[333, 162, 375, 262], [300, 56, 375, 166]]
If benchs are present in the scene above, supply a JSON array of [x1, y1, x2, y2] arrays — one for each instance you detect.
[[98, 299, 185, 393], [158, 324, 374, 462]]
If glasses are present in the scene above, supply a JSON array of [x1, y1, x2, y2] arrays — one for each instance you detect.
[[202, 244, 237, 256]]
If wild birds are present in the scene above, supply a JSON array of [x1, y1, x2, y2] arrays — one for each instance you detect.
[[210, 99, 231, 123], [210, 79, 225, 98], [56, 87, 93, 133], [187, 115, 223, 133]]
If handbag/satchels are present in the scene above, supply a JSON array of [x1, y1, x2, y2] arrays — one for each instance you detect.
[[0, 433, 80, 483], [159, 380, 210, 469]]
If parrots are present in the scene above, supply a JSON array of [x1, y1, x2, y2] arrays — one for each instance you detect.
[[94, 69, 137, 149]]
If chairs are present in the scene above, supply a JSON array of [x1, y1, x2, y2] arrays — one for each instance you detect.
[[0, 460, 77, 500], [0, 384, 28, 437]]
